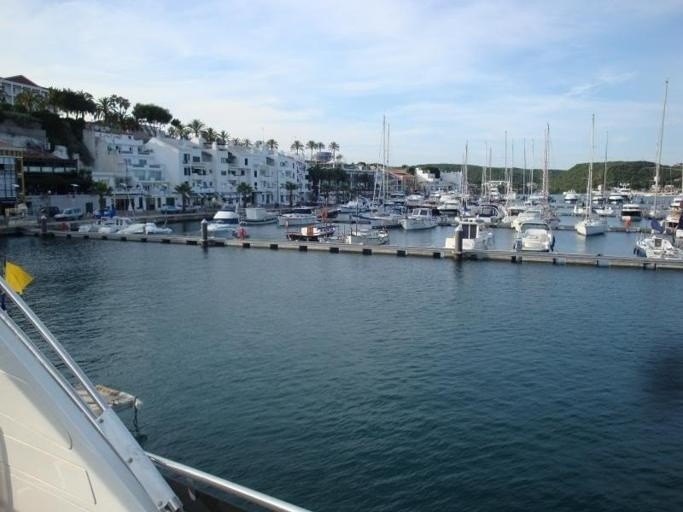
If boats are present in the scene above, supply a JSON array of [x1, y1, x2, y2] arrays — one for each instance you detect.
[[0, 273, 314, 512], [78, 215, 173, 235]]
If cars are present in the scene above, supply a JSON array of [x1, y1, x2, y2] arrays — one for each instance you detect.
[[160, 206, 179, 214], [37, 206, 59, 217], [53, 208, 83, 220], [175, 204, 196, 213]]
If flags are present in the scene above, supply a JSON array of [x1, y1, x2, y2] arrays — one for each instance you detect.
[[3, 260, 35, 296]]
